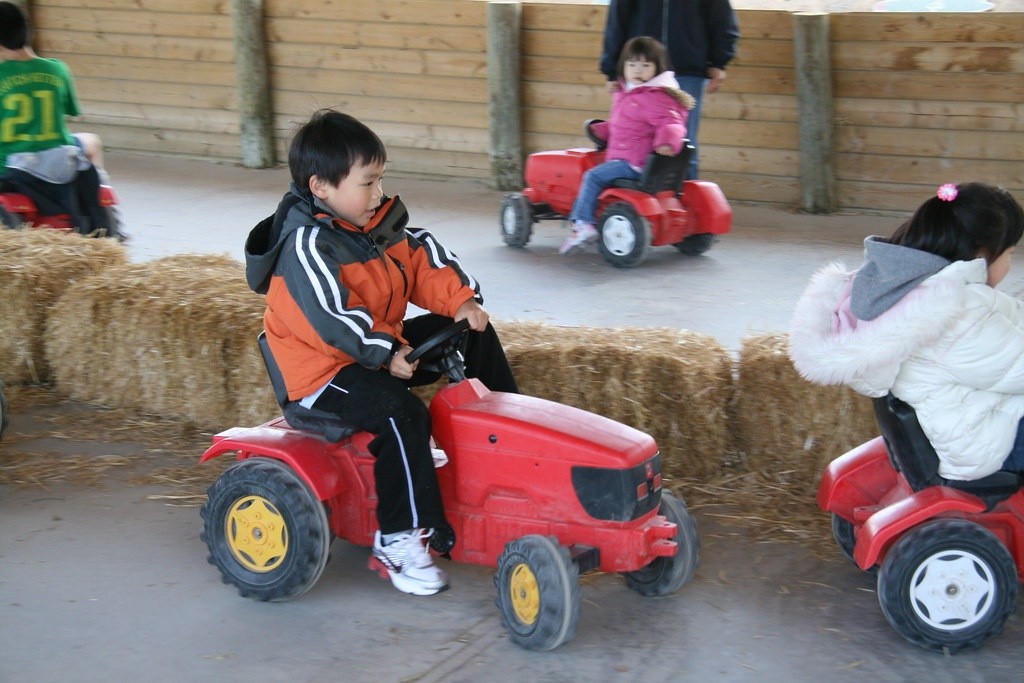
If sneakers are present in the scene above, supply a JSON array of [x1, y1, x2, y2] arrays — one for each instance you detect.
[[560, 223, 600, 256], [366, 526, 452, 597]]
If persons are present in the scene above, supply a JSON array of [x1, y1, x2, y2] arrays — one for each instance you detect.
[[0, 0, 110, 185], [852, 178, 1024, 492], [556, 34, 696, 256], [598, 1, 739, 181], [244, 109, 521, 597]]
